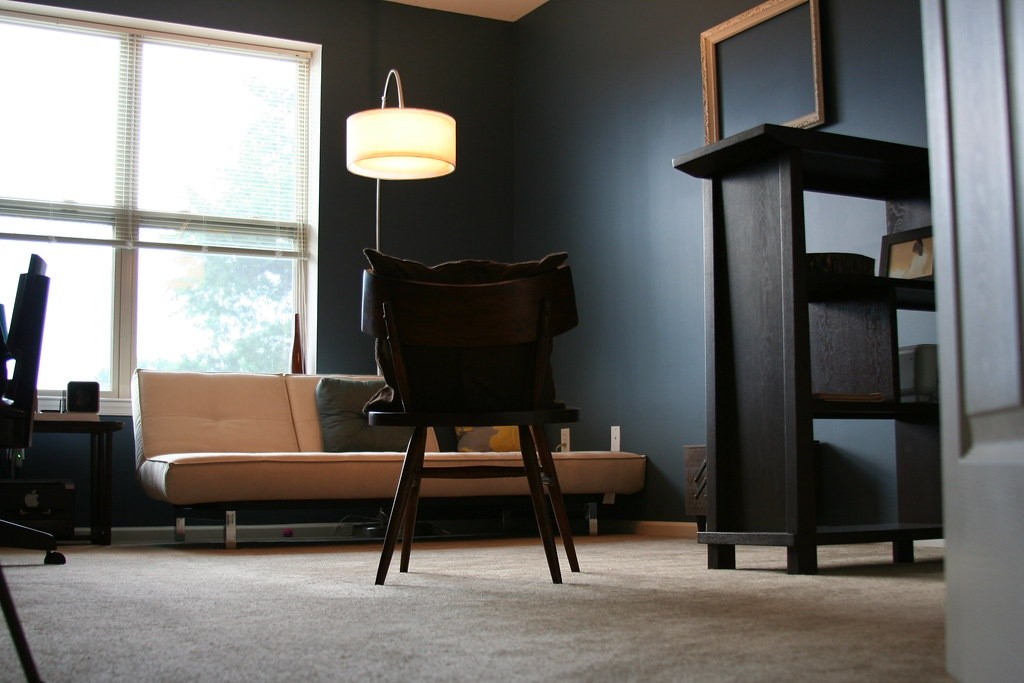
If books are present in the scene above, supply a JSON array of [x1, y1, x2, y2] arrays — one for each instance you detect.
[[34, 412, 101, 422]]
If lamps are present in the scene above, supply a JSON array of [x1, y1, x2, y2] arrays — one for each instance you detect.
[[346, 69, 457, 251]]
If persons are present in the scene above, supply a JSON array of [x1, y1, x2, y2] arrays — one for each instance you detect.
[[906, 240, 927, 278]]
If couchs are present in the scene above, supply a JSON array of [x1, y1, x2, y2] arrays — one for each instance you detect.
[[131, 369, 648, 550]]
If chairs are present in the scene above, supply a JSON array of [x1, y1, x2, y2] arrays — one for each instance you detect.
[[0, 254, 50, 683], [360, 246, 584, 585]]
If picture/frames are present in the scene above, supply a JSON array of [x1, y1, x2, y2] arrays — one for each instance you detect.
[[699, 1, 825, 147], [880, 226, 934, 282]]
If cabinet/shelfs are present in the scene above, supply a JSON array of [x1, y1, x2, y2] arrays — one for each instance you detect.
[[672, 122, 943, 575]]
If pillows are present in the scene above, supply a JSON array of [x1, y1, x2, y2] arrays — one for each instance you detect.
[[360, 249, 569, 412], [455, 425, 522, 451], [315, 378, 414, 452]]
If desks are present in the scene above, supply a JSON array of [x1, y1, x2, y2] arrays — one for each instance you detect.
[[685, 440, 824, 543], [0, 421, 126, 547]]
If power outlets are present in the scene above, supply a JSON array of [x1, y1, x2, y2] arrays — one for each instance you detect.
[[560, 428, 570, 452]]
